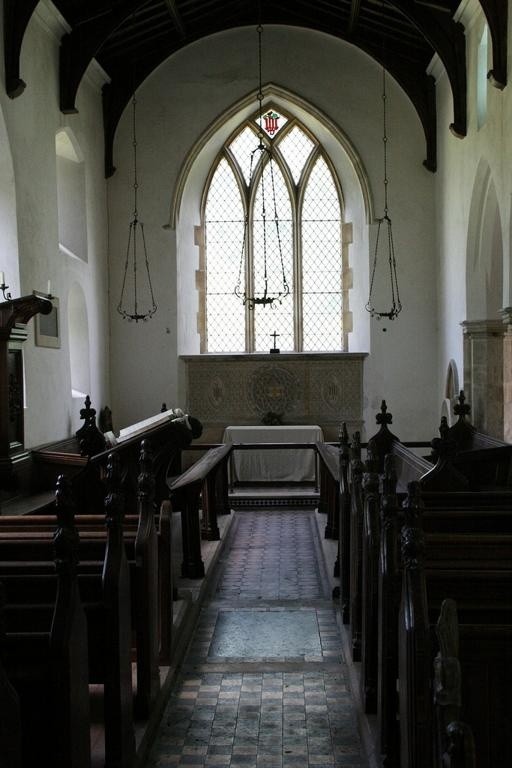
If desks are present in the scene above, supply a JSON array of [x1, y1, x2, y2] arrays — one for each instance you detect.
[[225, 425, 321, 482]]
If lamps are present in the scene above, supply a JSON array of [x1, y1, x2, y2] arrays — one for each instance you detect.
[[236, 26, 289, 309], [118, 90, 158, 323], [365, 68, 402, 320]]
